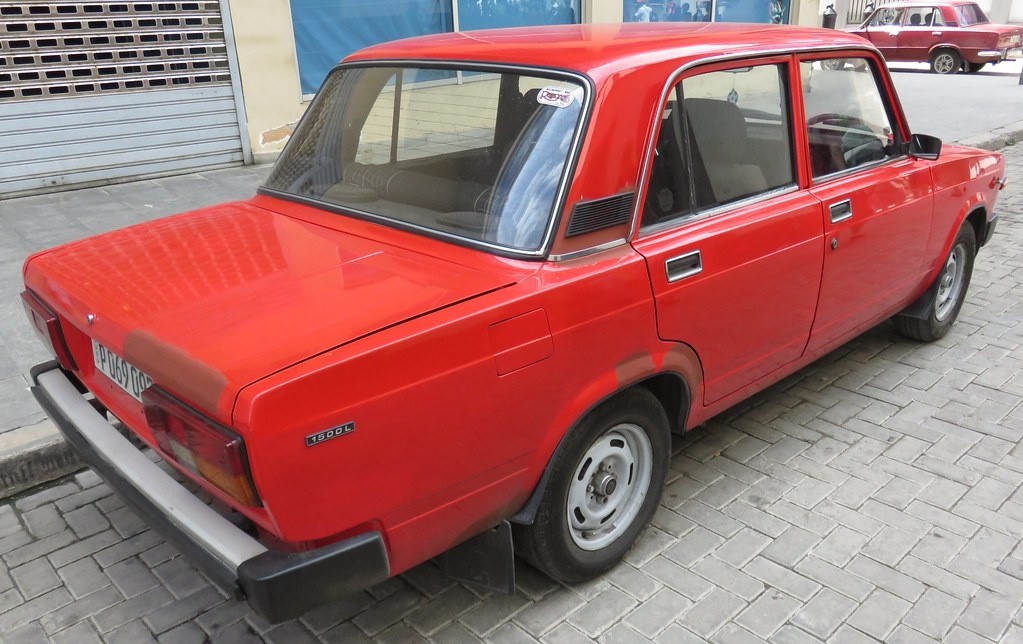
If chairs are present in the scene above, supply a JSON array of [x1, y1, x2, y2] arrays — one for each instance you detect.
[[499, 87, 580, 174], [910, 13, 938, 25], [667, 97, 768, 204]]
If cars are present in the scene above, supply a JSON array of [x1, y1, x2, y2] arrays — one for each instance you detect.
[[16, 21, 1009, 627], [818, 1, 1023, 76]]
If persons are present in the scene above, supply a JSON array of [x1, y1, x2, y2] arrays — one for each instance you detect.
[[883, 125, 894, 144], [840, 104, 886, 168]]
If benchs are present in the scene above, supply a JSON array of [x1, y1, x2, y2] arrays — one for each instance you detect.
[[340, 161, 554, 218]]
